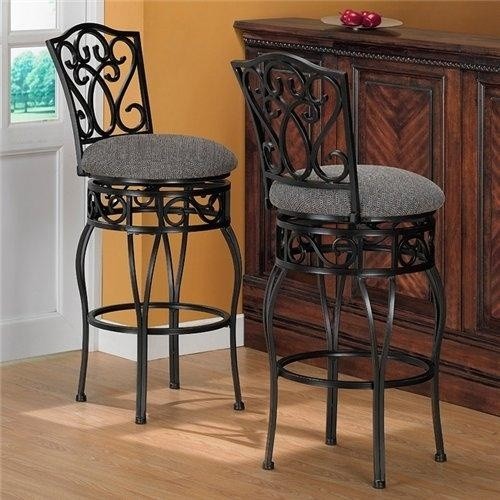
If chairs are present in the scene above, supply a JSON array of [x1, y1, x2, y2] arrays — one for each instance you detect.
[[229, 51, 446, 490], [46, 22, 244, 425]]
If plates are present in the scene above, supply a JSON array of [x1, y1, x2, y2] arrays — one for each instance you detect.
[[321, 15, 403, 30]]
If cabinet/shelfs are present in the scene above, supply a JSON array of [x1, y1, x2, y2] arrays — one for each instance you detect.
[[231, 17, 500, 420]]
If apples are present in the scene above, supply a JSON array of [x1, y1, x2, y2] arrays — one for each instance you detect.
[[339, 10, 381, 27]]
